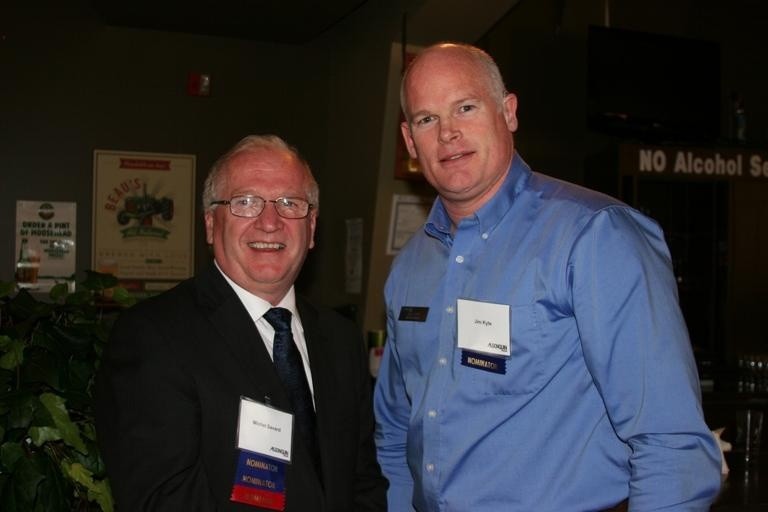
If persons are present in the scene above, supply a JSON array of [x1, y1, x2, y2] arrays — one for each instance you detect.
[[93, 135, 389, 510], [371, 41, 722, 512]]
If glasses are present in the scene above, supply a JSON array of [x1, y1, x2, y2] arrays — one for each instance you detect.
[[209, 193, 314, 220]]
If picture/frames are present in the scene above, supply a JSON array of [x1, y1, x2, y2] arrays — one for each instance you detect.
[[91, 147, 198, 282]]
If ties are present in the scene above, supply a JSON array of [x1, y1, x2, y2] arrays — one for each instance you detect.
[[263, 307, 326, 493]]
